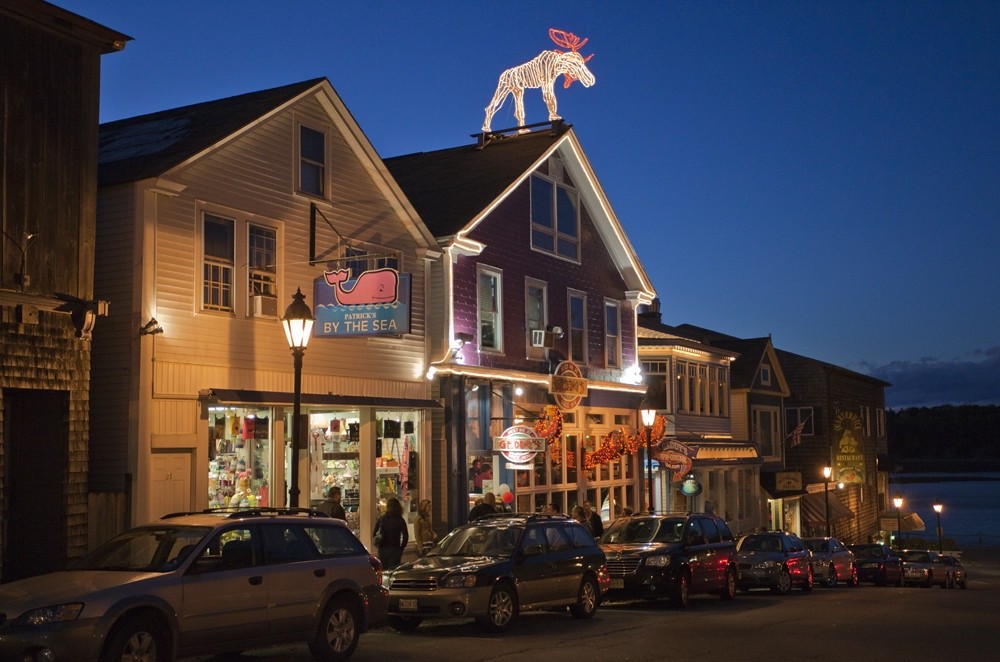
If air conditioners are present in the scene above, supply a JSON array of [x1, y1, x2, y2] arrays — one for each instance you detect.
[[530, 329, 554, 349], [249, 295, 278, 320]]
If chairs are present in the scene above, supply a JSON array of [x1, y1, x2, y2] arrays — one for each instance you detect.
[[223, 540, 254, 567], [174, 544, 199, 575]]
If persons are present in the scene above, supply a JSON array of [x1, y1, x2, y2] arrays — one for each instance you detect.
[[373, 498, 409, 572], [571, 505, 592, 546], [546, 503, 571, 550], [618, 507, 636, 543], [474, 464, 492, 489], [517, 460, 559, 487], [583, 501, 604, 541], [468, 492, 498, 524], [469, 459, 481, 480], [315, 487, 346, 554], [414, 500, 437, 558]]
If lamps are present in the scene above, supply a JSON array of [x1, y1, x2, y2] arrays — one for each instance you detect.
[[451, 332, 474, 359], [139, 318, 163, 336]]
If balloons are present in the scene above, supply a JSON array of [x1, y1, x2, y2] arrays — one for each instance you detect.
[[499, 484, 510, 495], [502, 492, 514, 504]]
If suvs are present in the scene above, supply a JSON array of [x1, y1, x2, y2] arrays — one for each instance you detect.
[[0, 506, 390, 662], [595, 510, 742, 610], [379, 512, 611, 634]]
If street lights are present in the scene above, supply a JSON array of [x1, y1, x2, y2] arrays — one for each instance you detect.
[[932, 499, 944, 553], [279, 287, 316, 510], [821, 459, 833, 537], [639, 388, 657, 515], [892, 489, 904, 548]]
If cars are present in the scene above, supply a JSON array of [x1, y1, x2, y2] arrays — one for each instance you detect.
[[802, 536, 861, 589], [734, 530, 814, 596], [844, 543, 906, 588], [901, 550, 969, 590]]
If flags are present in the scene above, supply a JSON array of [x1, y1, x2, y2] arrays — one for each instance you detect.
[[791, 415, 810, 448]]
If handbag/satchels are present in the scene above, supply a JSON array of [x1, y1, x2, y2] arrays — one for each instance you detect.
[[372, 515, 383, 547]]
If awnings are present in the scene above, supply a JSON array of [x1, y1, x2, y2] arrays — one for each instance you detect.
[[879, 512, 925, 532], [802, 482, 855, 526]]
